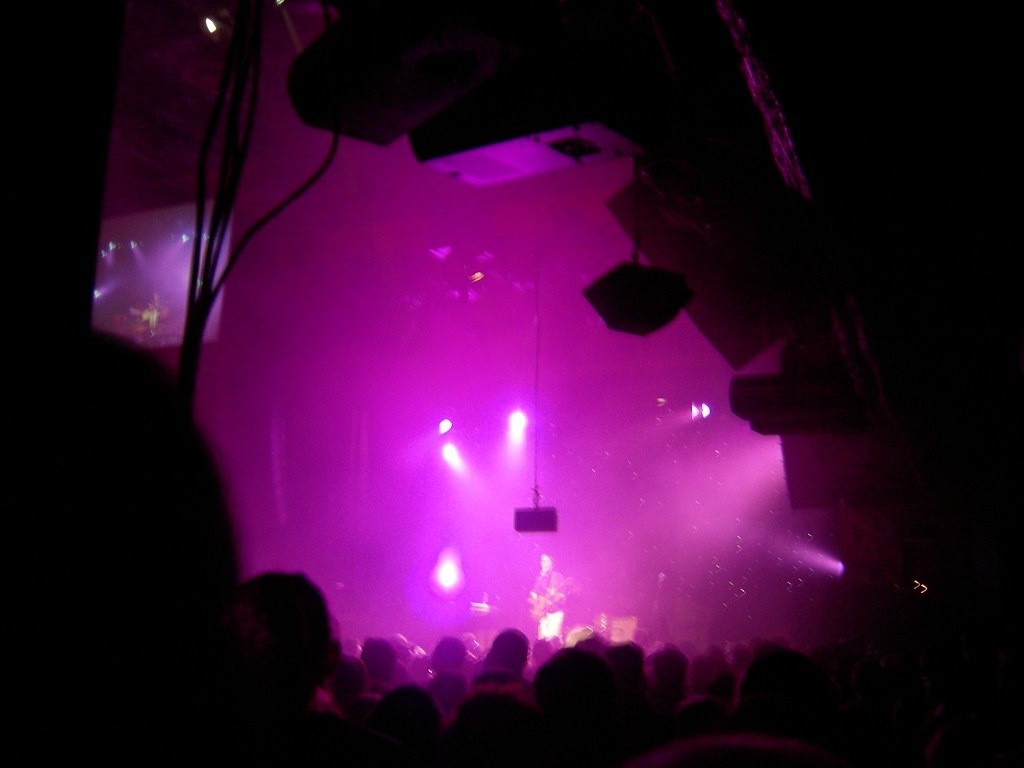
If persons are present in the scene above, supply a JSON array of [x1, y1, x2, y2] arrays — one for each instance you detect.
[[529, 554, 567, 639], [0, 315, 1024, 768]]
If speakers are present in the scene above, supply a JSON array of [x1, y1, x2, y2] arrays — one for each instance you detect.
[[586, 264, 693, 333], [288, 0, 516, 144], [516, 507, 558, 531]]
